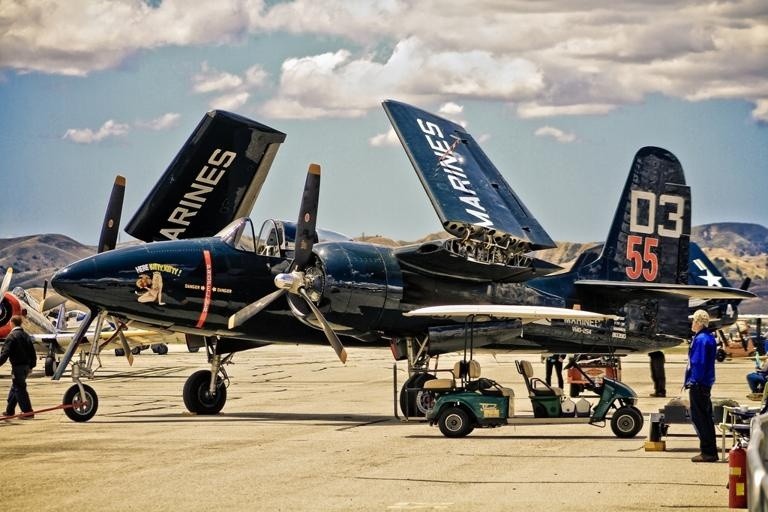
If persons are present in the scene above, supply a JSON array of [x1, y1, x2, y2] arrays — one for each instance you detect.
[[541, 352, 566, 391], [684, 309, 720, 462], [747, 359, 768, 392], [648, 352, 666, 397], [0, 316, 37, 420]]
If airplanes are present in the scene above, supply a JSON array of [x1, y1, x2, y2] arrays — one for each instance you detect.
[[0, 264, 187, 381], [43, 97, 761, 417]]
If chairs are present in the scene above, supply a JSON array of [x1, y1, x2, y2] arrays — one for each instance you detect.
[[264, 227, 282, 247]]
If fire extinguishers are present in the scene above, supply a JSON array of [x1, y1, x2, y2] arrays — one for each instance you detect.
[[726, 439, 747, 508]]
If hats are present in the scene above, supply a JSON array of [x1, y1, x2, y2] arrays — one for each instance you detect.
[[688, 309, 709, 324]]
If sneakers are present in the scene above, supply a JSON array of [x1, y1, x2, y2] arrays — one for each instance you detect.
[[692, 453, 718, 461]]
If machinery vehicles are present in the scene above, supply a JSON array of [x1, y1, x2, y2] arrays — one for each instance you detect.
[[716, 317, 768, 362], [563, 349, 624, 401]]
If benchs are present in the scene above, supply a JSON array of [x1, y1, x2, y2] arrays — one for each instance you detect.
[[467, 358, 516, 399], [422, 358, 465, 392], [519, 358, 565, 398]]
